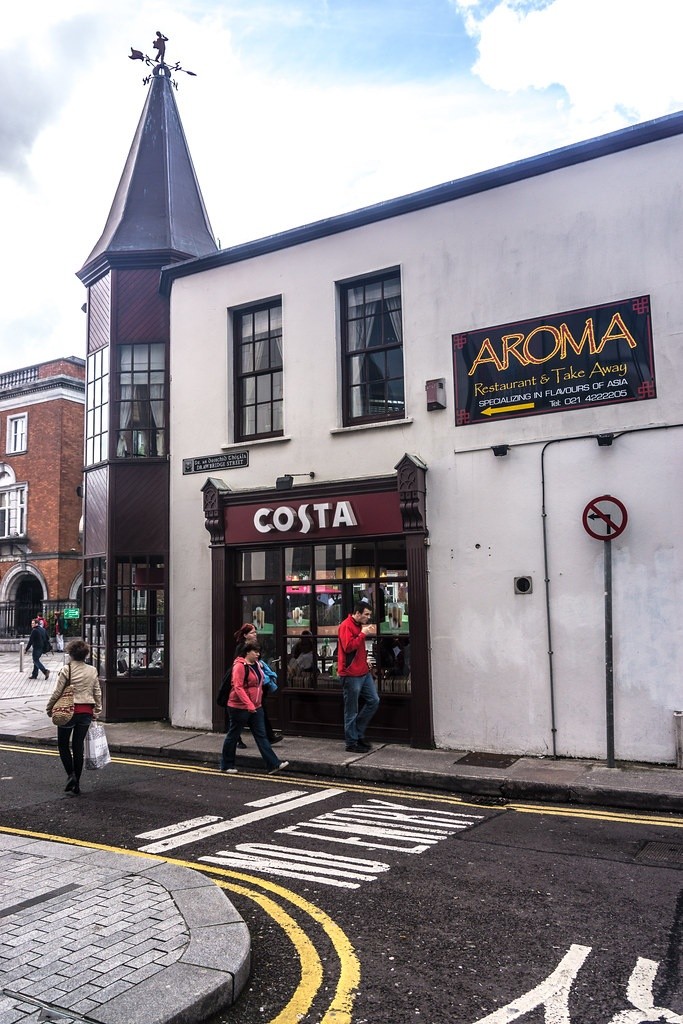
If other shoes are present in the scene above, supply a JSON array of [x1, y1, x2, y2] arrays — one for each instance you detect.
[[346, 738, 376, 753], [43, 670, 50, 680], [29, 675, 37, 679]]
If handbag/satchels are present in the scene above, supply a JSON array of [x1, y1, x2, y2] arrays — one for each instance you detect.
[[43, 639, 52, 654], [84, 720, 112, 771], [50, 685, 75, 726]]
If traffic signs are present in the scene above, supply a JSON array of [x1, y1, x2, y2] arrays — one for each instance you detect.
[[64, 608, 79, 618]]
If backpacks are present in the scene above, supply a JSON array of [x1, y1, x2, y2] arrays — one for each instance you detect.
[[217, 656, 249, 709]]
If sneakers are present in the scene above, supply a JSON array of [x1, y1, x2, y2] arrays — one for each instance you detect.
[[63, 775, 81, 796], [221, 768, 238, 774], [234, 739, 246, 748], [268, 731, 283, 744], [267, 760, 289, 774]]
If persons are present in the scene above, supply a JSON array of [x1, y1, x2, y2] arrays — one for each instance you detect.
[[220, 640, 290, 775], [337, 601, 380, 753], [289, 630, 318, 671], [46, 642, 103, 795], [35, 612, 55, 657], [230, 624, 284, 750], [23, 620, 53, 680]]
